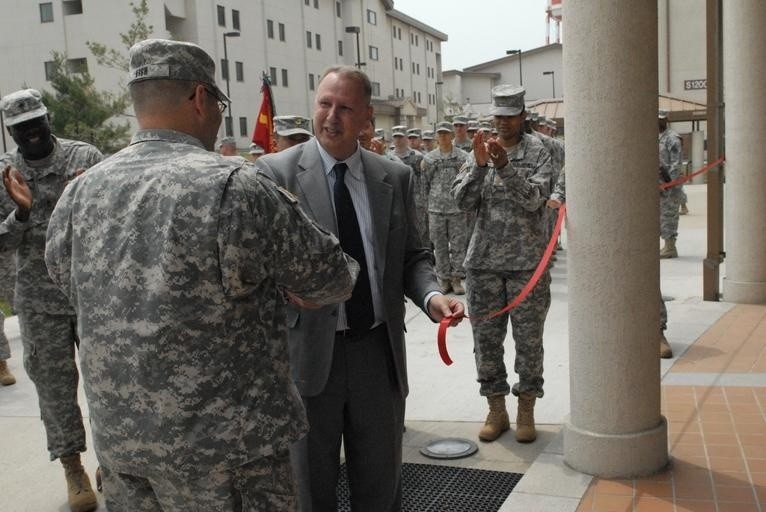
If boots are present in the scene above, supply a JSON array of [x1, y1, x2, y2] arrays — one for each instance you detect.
[[514, 393, 537, 444], [659, 238, 679, 259], [679, 204, 689, 215], [478, 393, 511, 442], [439, 278, 465, 294], [60, 455, 101, 512]]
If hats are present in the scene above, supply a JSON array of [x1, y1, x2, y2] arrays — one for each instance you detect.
[[273, 115, 316, 140], [489, 84, 526, 116], [247, 142, 265, 154], [658, 108, 669, 119], [1, 87, 50, 129], [372, 109, 559, 143], [127, 37, 233, 102], [218, 136, 237, 150]]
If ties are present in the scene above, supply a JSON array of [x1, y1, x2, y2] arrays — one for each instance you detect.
[[332, 162, 377, 344]]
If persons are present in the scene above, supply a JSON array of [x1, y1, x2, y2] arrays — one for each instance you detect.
[[0, 250, 22, 386], [255, 63, 466, 511], [213, 108, 690, 360], [0, 88, 107, 512], [448, 84, 556, 443], [42, 38, 363, 510]]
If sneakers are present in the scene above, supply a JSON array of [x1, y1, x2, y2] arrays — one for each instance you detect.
[[660, 330, 672, 359], [0, 360, 16, 386], [550, 242, 564, 262]]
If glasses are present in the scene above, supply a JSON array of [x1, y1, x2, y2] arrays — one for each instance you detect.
[[189, 85, 228, 114]]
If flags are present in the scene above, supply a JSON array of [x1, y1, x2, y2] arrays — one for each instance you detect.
[[249, 72, 276, 156]]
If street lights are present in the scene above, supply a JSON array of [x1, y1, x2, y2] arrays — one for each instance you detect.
[[543, 70, 555, 98], [345, 25, 360, 69], [222, 30, 240, 137], [506, 49, 522, 86], [434, 81, 444, 123]]
